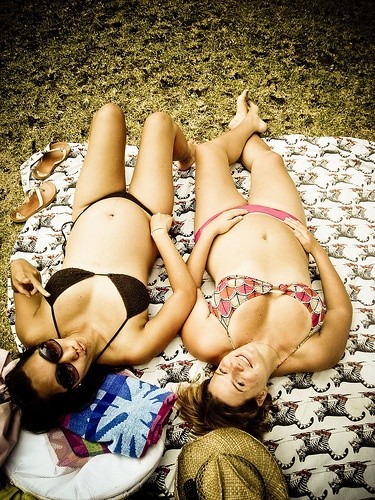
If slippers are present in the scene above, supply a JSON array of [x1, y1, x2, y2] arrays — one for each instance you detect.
[[9, 181, 58, 223], [32, 142, 71, 180]]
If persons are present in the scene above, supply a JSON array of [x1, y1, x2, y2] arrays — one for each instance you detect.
[[4, 101, 202, 437], [176, 88, 353, 434]]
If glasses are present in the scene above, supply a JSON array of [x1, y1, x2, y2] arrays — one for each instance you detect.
[[38, 338, 79, 389]]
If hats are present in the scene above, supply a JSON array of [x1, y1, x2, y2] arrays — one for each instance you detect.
[[175, 427, 290, 500]]
